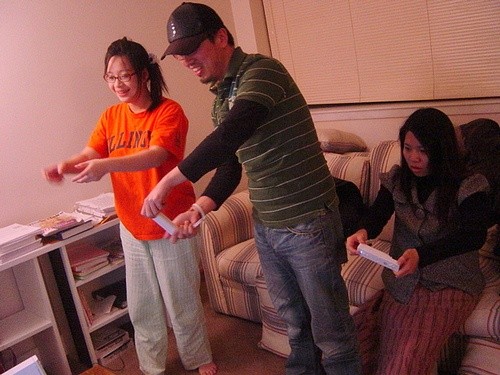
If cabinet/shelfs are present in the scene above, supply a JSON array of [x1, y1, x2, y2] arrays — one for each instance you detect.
[[47, 217, 133, 367], [0, 217, 75, 374]]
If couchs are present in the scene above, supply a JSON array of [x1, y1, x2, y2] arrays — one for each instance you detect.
[[198, 139, 500, 375]]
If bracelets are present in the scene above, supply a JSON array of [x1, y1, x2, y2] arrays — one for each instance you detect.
[[187, 199, 208, 227]]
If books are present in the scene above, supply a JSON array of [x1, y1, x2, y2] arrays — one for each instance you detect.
[[76, 287, 94, 329], [41, 211, 95, 241], [74, 192, 115, 218], [0, 223, 44, 266], [65, 242, 110, 280], [103, 238, 125, 260], [27, 211, 79, 238]]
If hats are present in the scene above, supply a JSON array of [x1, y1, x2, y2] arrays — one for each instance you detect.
[[161, 2, 223, 60]]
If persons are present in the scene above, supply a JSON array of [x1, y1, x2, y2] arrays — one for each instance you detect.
[[41, 36, 218, 375], [346, 107, 491, 375], [140, 2, 363, 375]]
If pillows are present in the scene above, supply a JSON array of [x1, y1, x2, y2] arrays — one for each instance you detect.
[[316, 128, 368, 153], [253, 276, 292, 360]]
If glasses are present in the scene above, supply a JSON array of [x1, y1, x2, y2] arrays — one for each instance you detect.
[[104, 71, 135, 83]]
